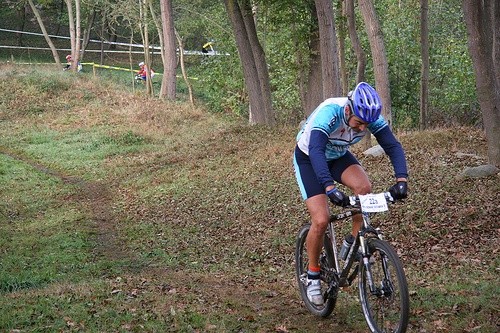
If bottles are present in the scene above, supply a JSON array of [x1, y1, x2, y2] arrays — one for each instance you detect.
[[340, 235, 355, 261]]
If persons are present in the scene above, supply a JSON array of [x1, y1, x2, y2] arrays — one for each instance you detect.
[[293, 82, 408, 310], [134, 62, 156, 82], [63, 55, 82, 73], [201, 40, 215, 65]]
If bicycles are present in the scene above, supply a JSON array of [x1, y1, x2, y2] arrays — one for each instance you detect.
[[294, 191, 410, 333]]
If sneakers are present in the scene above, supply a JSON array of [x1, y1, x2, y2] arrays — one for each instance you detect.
[[305, 274, 325, 310], [350, 247, 376, 263]]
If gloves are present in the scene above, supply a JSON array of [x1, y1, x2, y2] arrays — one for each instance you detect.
[[388, 181, 408, 200], [327, 187, 350, 207]]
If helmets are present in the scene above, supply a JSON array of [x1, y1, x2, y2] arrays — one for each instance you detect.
[[66, 54, 73, 60], [138, 61, 145, 67], [348, 82, 382, 123]]
